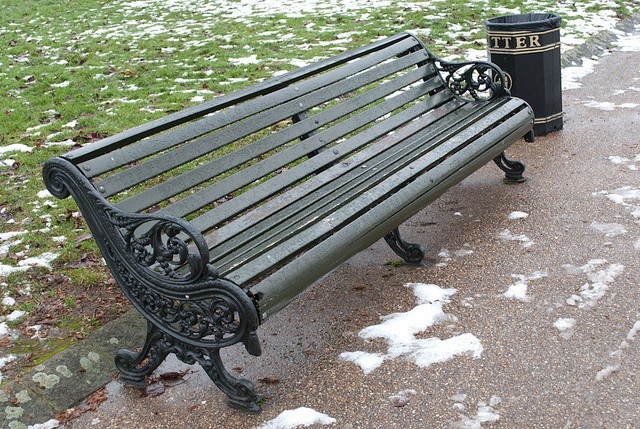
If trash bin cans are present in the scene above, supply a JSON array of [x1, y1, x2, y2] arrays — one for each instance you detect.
[[484, 13, 563, 136]]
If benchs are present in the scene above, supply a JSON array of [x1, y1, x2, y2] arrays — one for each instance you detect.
[[43, 32, 534, 414]]
